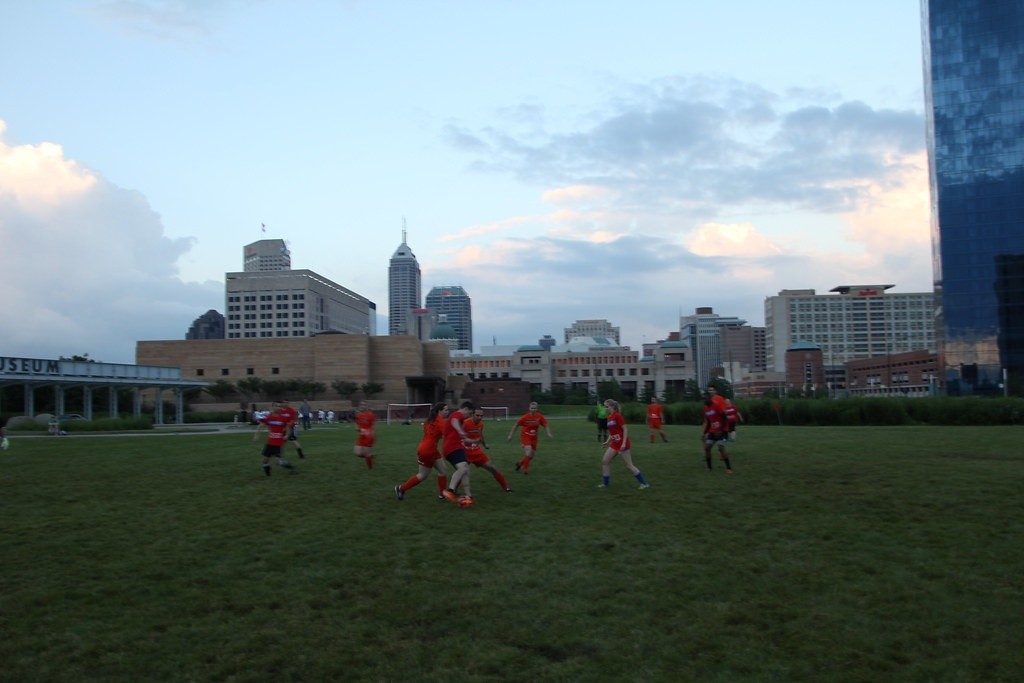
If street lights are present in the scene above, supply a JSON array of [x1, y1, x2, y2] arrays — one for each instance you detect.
[[824, 348, 835, 399]]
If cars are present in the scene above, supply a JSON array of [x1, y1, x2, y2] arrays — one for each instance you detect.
[[52, 413, 88, 421]]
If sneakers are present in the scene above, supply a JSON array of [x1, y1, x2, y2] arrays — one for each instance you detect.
[[438, 495, 446, 500], [443, 489, 457, 502], [395, 485, 404, 501]]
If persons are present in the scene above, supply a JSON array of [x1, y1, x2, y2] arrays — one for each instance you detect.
[[594, 398, 609, 442], [253, 400, 294, 476], [352, 399, 377, 469], [393, 400, 513, 508], [507, 402, 553, 474], [0, 432, 9, 450], [299, 399, 312, 430], [253, 406, 335, 425], [595, 399, 650, 490], [281, 397, 305, 459], [702, 385, 744, 474], [646, 396, 668, 443], [48, 417, 58, 433]]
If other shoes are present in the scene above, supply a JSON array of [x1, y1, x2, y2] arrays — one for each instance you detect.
[[515, 462, 521, 471], [465, 496, 473, 501], [523, 471, 528, 475], [505, 488, 512, 492], [720, 453, 724, 461], [638, 484, 650, 490], [727, 469, 732, 473], [597, 484, 606, 488]]
